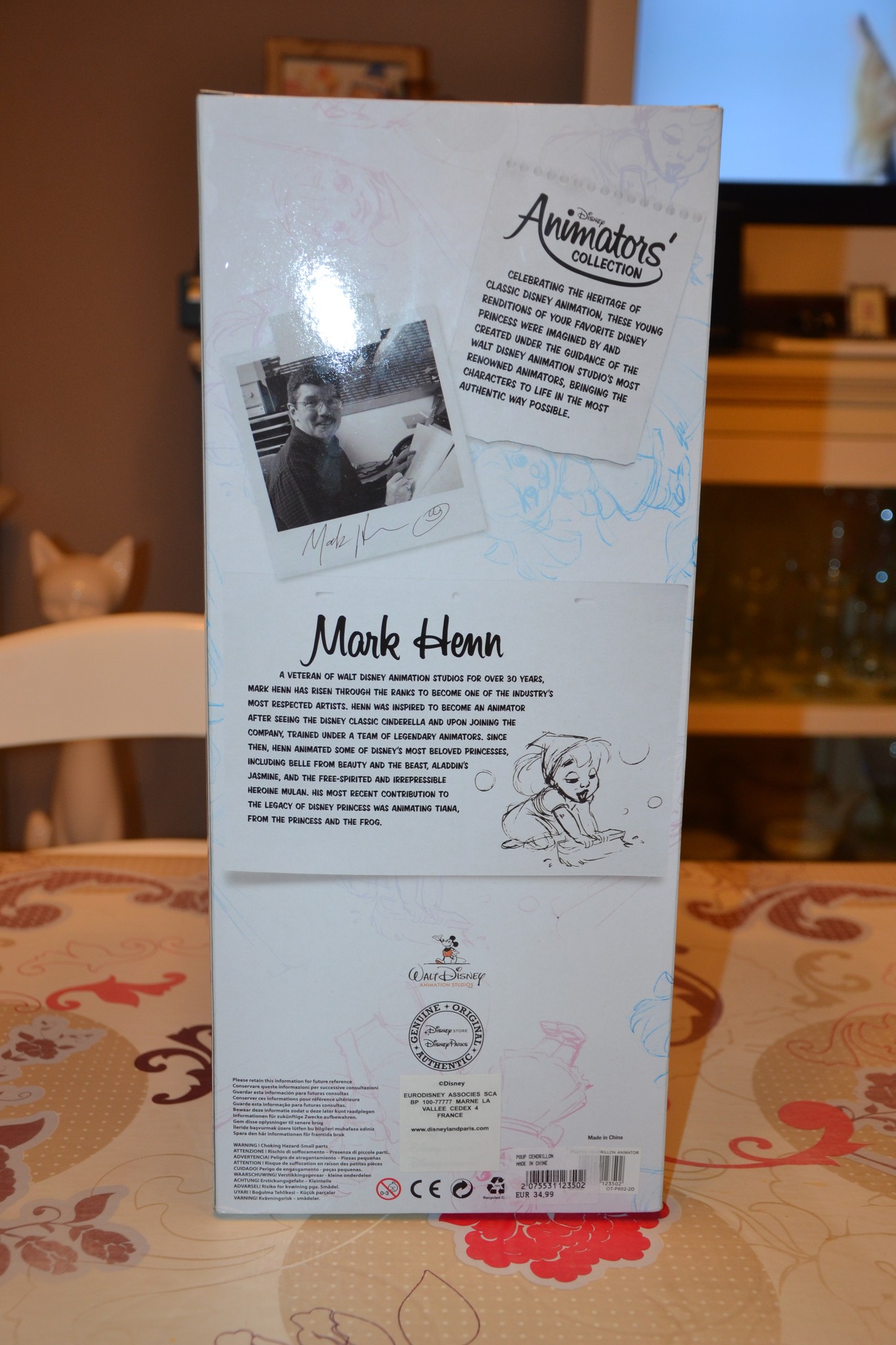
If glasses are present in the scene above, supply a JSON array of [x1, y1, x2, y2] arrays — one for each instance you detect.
[[294, 397, 344, 411]]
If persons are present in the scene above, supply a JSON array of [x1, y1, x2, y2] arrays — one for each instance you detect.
[[268, 365, 417, 531]]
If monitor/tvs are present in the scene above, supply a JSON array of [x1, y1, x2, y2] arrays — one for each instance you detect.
[[580, 1, 896, 229]]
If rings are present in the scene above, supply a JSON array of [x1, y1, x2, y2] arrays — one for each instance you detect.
[[404, 483, 410, 489]]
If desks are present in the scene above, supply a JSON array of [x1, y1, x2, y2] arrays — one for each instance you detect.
[[0, 832, 896, 1345]]
